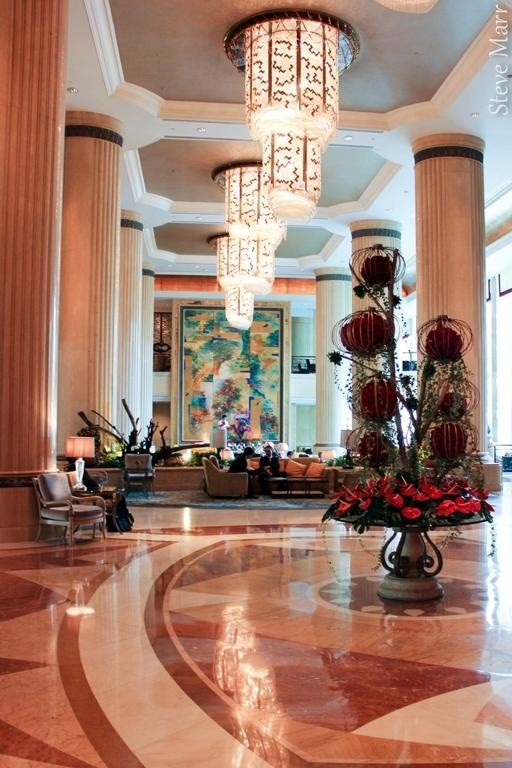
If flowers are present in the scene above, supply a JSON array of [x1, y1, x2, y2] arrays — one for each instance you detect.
[[320, 470, 501, 573]]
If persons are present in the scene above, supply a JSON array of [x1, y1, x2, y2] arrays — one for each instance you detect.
[[227, 446, 262, 500], [217, 414, 232, 431], [231, 418, 244, 442], [257, 445, 280, 497]]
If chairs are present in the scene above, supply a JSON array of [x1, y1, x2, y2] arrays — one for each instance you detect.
[[469, 451, 504, 493], [30, 468, 127, 561], [125, 453, 157, 501], [201, 455, 251, 503], [297, 359, 315, 374], [326, 463, 364, 496]]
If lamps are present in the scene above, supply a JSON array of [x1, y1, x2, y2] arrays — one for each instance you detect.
[[210, 160, 286, 296], [65, 436, 97, 491], [210, 232, 254, 330], [222, 7, 361, 224]]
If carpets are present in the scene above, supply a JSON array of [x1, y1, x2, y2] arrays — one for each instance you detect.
[[123, 486, 337, 510]]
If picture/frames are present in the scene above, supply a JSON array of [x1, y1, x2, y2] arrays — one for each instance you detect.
[[168, 297, 293, 448]]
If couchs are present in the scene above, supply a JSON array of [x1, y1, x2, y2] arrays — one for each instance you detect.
[[247, 456, 335, 497]]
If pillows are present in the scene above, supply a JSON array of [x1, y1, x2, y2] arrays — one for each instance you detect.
[[248, 459, 259, 470], [280, 458, 326, 479]]
